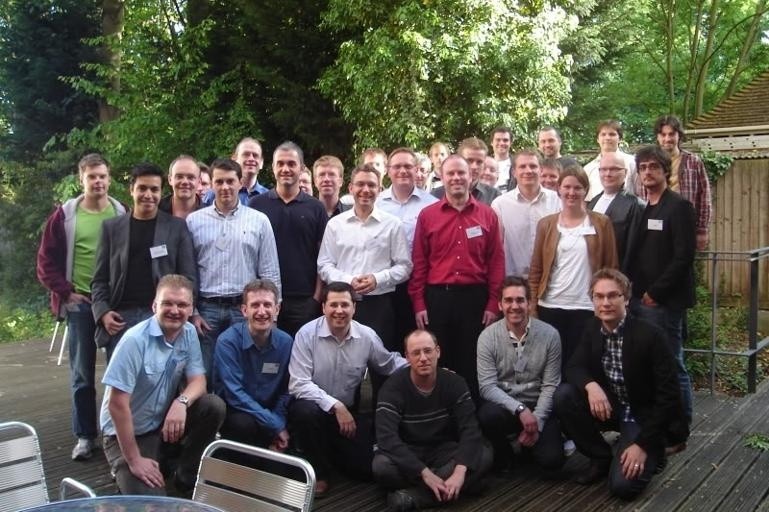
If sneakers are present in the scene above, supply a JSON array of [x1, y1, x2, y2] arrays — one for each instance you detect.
[[70, 437, 93, 460], [386, 489, 413, 512]]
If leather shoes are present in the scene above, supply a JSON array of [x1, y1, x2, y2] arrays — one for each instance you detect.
[[173, 474, 193, 494], [664, 441, 686, 454], [315, 480, 329, 493], [580, 464, 609, 483]]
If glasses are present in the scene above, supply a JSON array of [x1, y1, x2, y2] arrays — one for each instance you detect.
[[598, 167, 624, 172], [387, 164, 415, 170], [351, 181, 378, 188], [406, 344, 436, 357], [592, 291, 624, 299]]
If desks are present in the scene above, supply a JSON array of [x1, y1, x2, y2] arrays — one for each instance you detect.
[[17, 495, 226, 512]]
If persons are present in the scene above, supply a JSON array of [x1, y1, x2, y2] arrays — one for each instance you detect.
[[100, 274, 225, 496], [288, 282, 409, 498], [552, 268, 683, 499], [476, 275, 562, 473], [37, 115, 712, 459], [371, 328, 491, 508], [212, 280, 296, 473]]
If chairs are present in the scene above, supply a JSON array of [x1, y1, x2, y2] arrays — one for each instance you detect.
[[191, 438, 318, 512], [0, 420, 99, 512], [47, 320, 68, 366]]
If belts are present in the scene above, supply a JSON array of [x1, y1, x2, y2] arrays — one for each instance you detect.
[[199, 295, 241, 304], [356, 291, 395, 301], [441, 284, 473, 291]]
